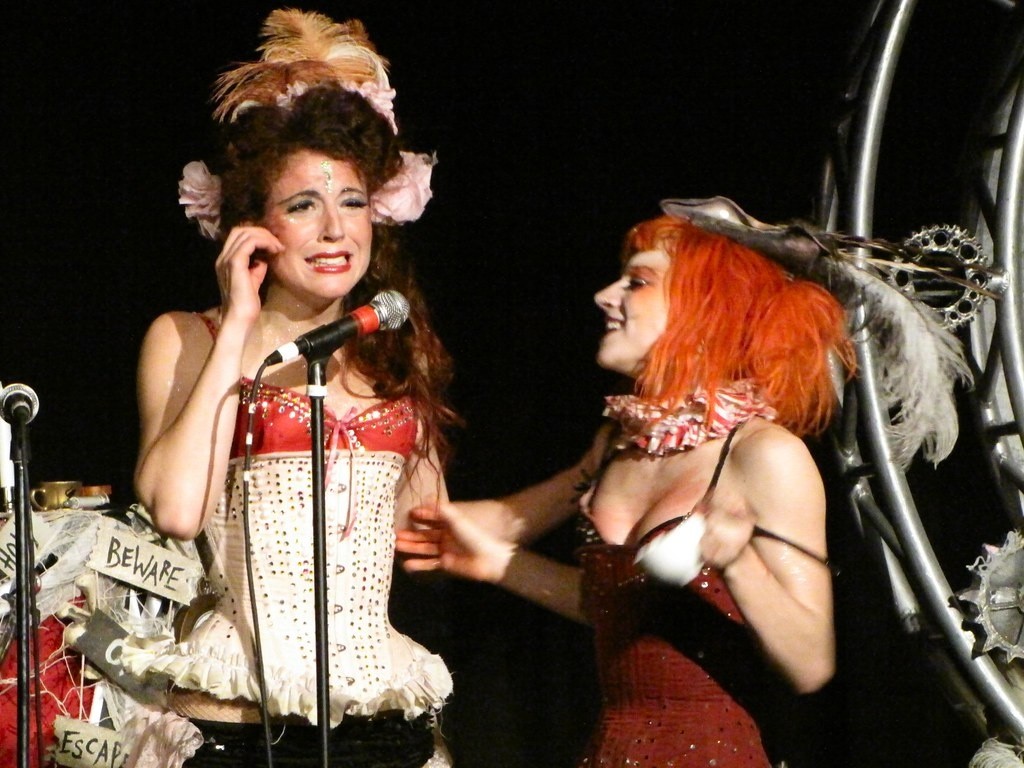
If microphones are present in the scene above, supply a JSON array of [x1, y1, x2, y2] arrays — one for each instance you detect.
[[0, 383, 41, 425], [264, 290, 410, 366]]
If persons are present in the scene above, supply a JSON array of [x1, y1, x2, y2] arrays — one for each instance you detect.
[[395, 217, 835, 768], [133, 83, 613, 768]]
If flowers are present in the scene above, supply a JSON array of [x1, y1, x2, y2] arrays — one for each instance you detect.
[[178, 80, 441, 241]]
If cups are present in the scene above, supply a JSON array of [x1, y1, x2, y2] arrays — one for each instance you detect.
[[76, 486, 112, 497], [29, 481, 78, 510]]
